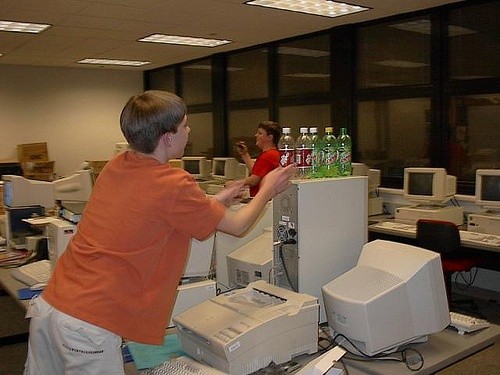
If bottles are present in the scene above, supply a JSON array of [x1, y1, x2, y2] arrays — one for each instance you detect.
[[278, 126, 352, 180]]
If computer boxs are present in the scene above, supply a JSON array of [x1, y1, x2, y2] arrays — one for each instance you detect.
[[368, 197, 383, 215], [468, 214, 500, 236], [395, 207, 463, 225], [47, 220, 77, 271], [274, 176, 369, 324]]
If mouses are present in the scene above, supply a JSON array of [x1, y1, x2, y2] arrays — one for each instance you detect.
[[30, 283, 47, 290]]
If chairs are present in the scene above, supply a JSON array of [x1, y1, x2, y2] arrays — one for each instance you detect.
[[416, 219, 480, 303]]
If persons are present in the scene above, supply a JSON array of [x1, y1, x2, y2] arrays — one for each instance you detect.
[[225, 121, 283, 197], [24, 90, 297, 375]]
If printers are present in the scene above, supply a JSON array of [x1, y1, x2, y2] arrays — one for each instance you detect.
[[175, 279, 320, 375]]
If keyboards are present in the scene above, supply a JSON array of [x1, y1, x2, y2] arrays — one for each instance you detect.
[[449, 312, 490, 335], [459, 231, 500, 246], [11, 260, 53, 286], [375, 222, 417, 234]]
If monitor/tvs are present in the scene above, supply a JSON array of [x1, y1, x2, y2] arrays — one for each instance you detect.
[[226, 226, 274, 288], [181, 157, 240, 180], [351, 163, 380, 193], [475, 169, 500, 217], [404, 168, 456, 210], [3, 169, 92, 215], [321, 240, 451, 356]]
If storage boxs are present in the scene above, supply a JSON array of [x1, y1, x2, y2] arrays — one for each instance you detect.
[[17, 142, 55, 181]]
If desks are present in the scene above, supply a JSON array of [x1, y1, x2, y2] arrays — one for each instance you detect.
[[0, 267, 32, 312], [368, 213, 500, 253]]
